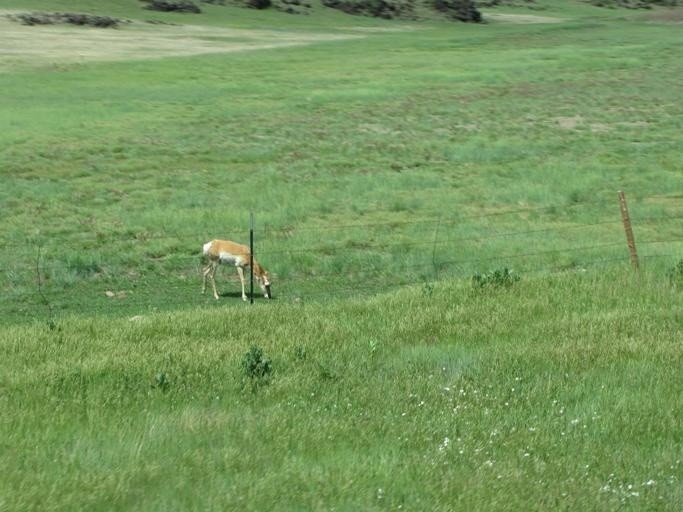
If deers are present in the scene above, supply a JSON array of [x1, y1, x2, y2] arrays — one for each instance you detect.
[[199, 239, 273, 302]]
